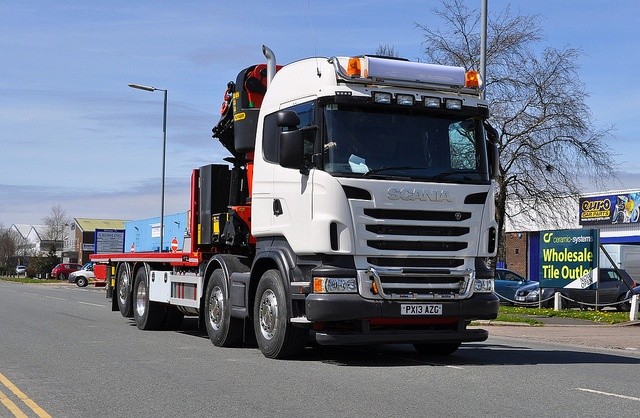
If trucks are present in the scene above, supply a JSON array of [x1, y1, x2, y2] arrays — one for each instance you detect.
[[89, 45, 499, 360]]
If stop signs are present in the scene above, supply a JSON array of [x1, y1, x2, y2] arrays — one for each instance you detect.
[[131, 245, 134, 253], [172, 239, 178, 251]]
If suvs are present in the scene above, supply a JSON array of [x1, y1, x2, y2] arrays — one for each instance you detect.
[[51, 263, 82, 280], [68, 262, 109, 287]]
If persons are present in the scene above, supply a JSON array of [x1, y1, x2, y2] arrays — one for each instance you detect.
[[410, 127, 443, 168]]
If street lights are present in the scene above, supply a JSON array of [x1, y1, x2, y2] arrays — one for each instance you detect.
[[127, 83, 167, 253]]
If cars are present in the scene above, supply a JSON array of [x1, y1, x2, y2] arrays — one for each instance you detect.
[[514, 268, 635, 310], [16, 265, 27, 274], [495, 268, 539, 303]]
